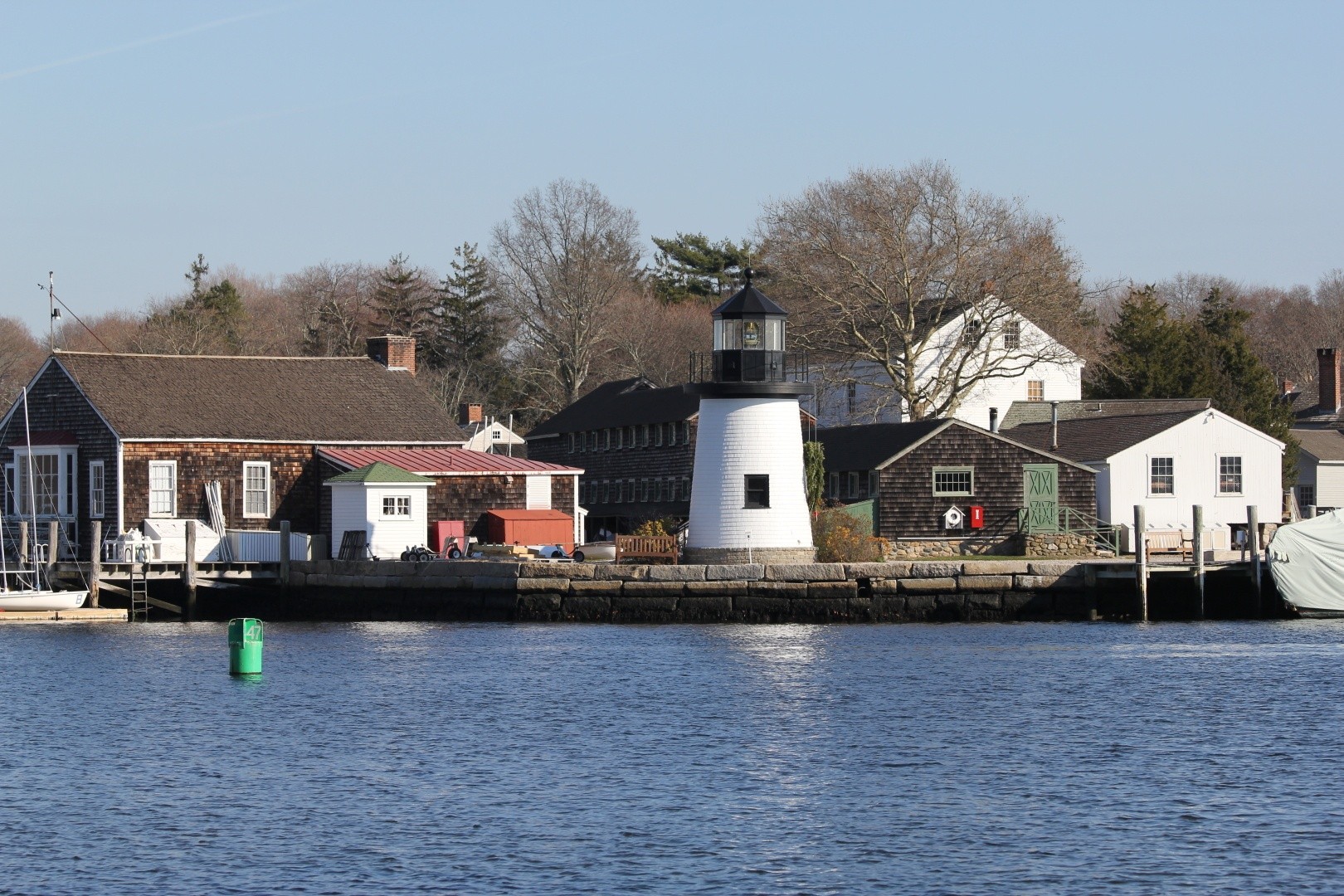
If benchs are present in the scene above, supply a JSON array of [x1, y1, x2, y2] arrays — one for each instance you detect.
[[1144, 528, 1194, 563], [614, 533, 680, 565]]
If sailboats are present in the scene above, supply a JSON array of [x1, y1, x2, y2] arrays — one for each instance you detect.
[[0, 386, 91, 611]]
